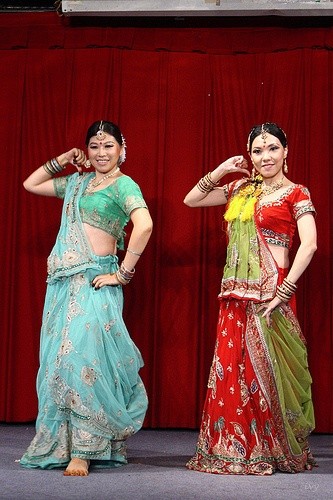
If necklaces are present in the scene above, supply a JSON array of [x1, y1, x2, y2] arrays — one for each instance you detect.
[[260, 176, 286, 196], [84, 167, 120, 193]]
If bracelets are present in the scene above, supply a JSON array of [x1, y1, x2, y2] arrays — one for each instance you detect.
[[116, 260, 135, 285], [196, 171, 220, 192], [275, 278, 297, 301], [126, 249, 142, 258], [43, 156, 66, 175]]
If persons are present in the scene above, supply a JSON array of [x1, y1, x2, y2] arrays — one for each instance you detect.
[[184, 122, 317, 476], [14, 120, 153, 476]]
